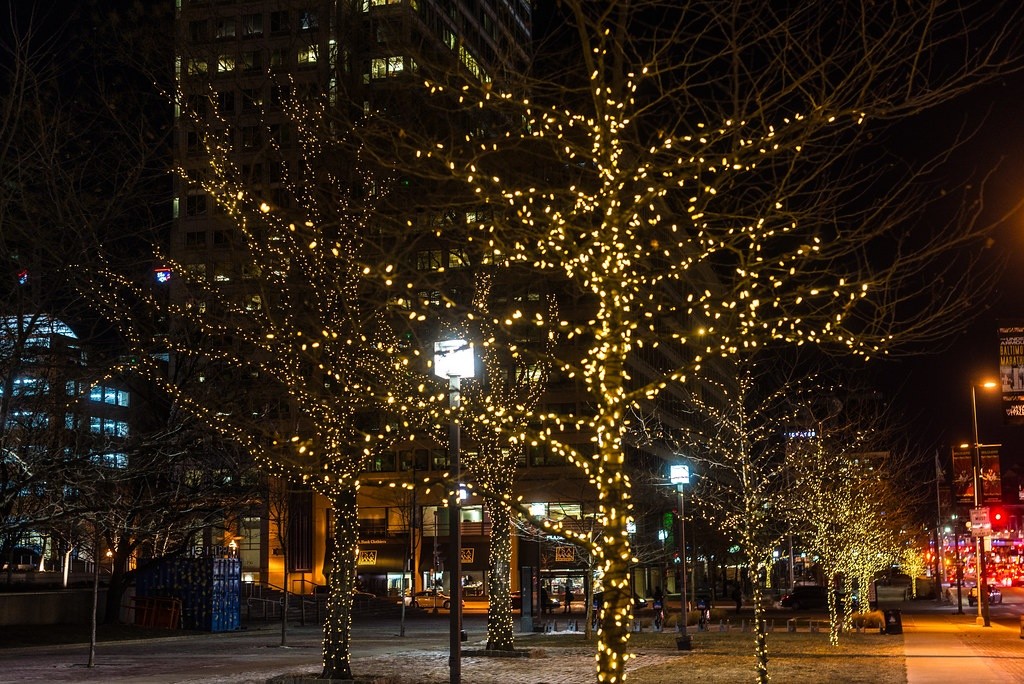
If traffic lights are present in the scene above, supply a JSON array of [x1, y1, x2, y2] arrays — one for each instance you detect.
[[995, 513, 1002, 520]]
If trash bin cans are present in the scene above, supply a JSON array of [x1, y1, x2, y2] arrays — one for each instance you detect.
[[882, 607, 903, 634]]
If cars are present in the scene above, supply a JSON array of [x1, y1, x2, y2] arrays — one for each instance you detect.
[[780, 585, 858, 612], [584, 589, 648, 610], [509, 591, 560, 611], [404, 590, 466, 610], [0, 548, 61, 572], [311, 585, 376, 600], [967, 584, 1002, 607]]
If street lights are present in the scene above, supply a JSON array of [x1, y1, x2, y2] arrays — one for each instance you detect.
[[670, 465, 693, 650], [434, 338, 475, 684], [971, 381, 997, 627]]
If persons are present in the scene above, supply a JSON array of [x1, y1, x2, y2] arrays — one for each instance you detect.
[[562, 587, 571, 613]]
